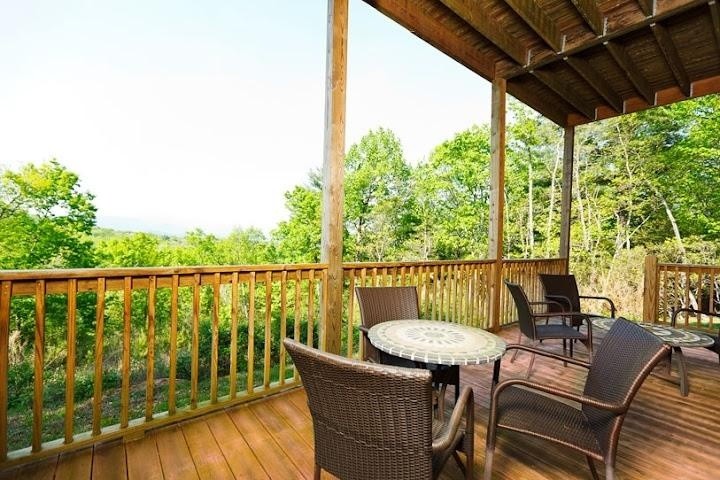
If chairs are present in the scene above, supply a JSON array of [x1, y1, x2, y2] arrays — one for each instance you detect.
[[671, 308, 720, 367], [281, 338, 478, 478], [482, 317, 670, 480], [354, 287, 451, 425], [504, 278, 593, 380], [538, 274, 615, 367]]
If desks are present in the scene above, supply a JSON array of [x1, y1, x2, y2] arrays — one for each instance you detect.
[[582, 318, 714, 397], [365, 317, 506, 414]]
